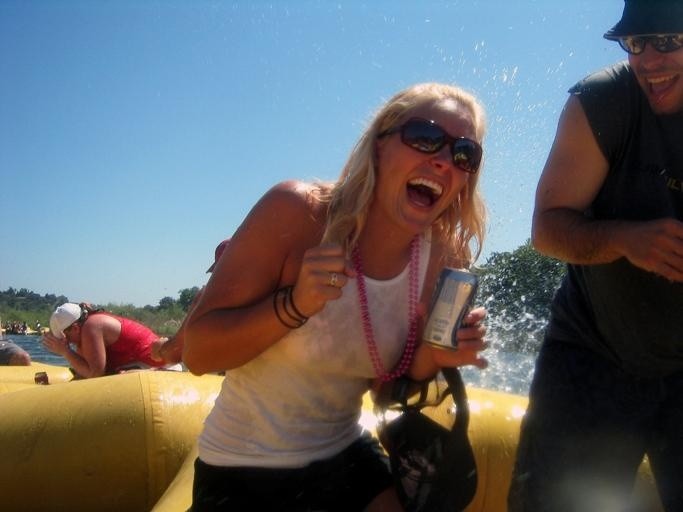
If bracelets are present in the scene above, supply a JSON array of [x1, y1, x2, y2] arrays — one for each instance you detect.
[[273, 286, 302, 329], [282, 285, 308, 326], [288, 283, 309, 320]]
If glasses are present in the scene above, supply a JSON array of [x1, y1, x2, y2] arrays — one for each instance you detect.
[[379, 116, 482, 173], [617, 35, 682, 54]]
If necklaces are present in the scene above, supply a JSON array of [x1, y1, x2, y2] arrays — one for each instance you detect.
[[350, 229, 421, 384]]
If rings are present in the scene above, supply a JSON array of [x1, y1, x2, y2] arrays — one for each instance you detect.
[[329, 271, 338, 287]]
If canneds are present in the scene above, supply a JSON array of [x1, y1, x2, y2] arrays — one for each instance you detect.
[[35, 372, 48, 384], [416, 265, 478, 351]]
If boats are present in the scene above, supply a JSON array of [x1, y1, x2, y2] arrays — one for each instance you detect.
[[2, 325, 51, 339], [150, 380, 662, 512], [1, 358, 225, 512]]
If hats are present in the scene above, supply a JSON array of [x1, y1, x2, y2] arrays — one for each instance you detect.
[[205, 239, 230, 272], [49, 303, 82, 339], [602, 0, 683, 40]]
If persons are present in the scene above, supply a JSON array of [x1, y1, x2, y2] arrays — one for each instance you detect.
[[39, 302, 183, 380], [179, 81, 491, 512], [2, 318, 40, 335], [506, 1, 681, 511], [0, 338, 30, 366], [149, 235, 233, 367]]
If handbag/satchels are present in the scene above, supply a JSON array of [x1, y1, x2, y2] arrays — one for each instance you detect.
[[380, 410, 477, 510]]
